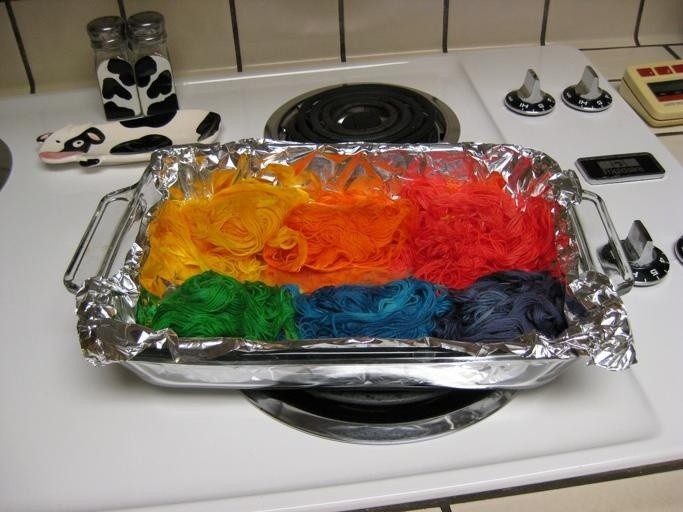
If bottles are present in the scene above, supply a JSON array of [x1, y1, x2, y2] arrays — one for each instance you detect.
[[127, 11, 178, 117], [87, 17, 142, 121]]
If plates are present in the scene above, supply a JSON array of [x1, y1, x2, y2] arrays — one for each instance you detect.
[[65, 139, 632, 394], [36, 109, 221, 170]]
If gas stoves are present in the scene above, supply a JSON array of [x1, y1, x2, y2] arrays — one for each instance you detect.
[[234, 385, 516, 443], [256, 78, 463, 179]]
